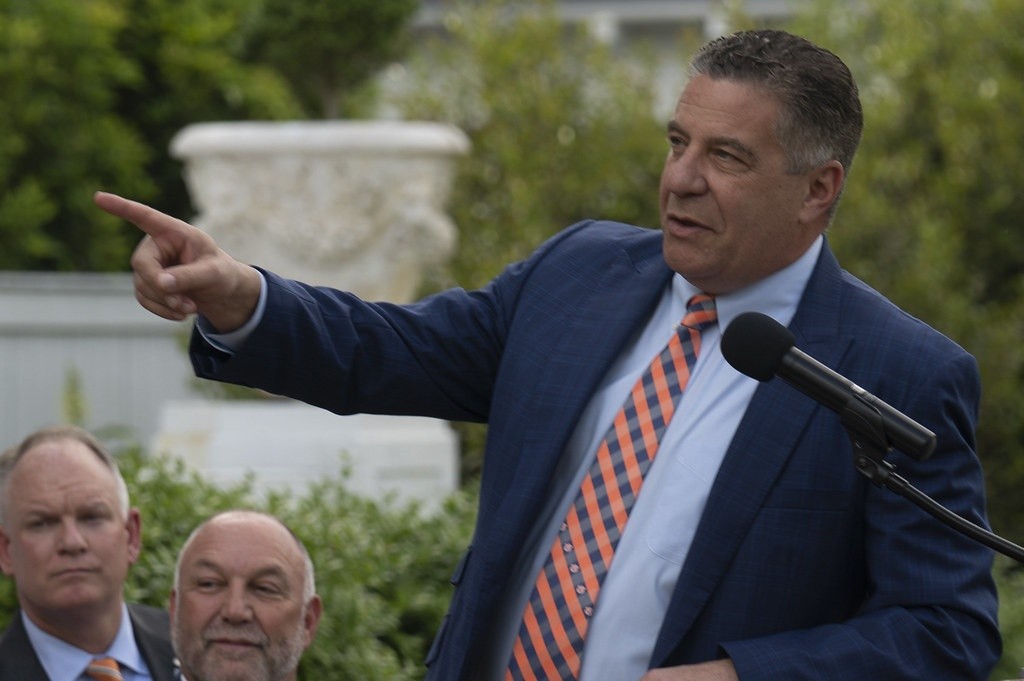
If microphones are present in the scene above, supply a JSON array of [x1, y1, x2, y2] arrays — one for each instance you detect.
[[720, 311, 938, 462]]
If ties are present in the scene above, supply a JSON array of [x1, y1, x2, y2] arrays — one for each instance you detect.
[[503, 292, 718, 681], [86, 657, 124, 681]]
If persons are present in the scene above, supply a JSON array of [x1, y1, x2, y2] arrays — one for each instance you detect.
[[169, 510, 323, 681], [0, 428, 177, 681], [95, 29, 1006, 681]]
